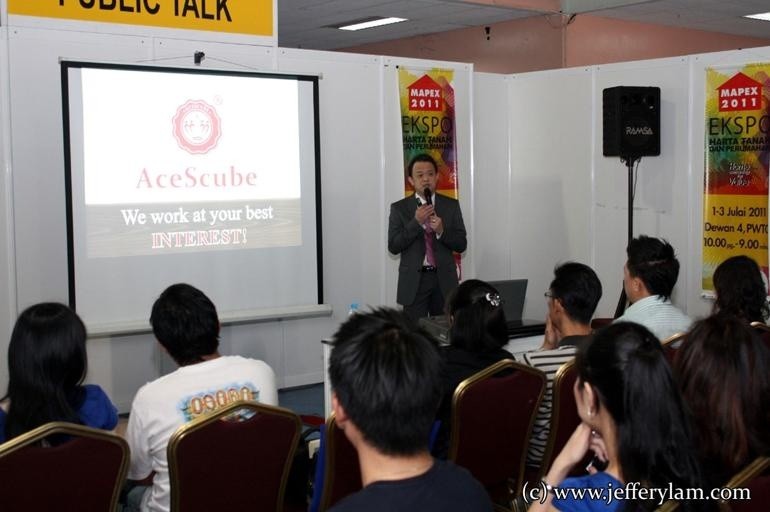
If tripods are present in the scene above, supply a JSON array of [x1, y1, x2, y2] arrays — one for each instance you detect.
[[613, 164, 645, 319]]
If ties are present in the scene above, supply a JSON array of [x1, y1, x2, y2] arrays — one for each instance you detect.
[[424, 201, 437, 267]]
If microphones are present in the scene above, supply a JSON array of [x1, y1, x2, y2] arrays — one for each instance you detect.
[[423, 187, 435, 215]]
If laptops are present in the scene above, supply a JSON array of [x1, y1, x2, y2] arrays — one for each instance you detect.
[[482, 278, 528, 322]]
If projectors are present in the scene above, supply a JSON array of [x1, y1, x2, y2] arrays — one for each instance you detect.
[[418, 315, 454, 345]]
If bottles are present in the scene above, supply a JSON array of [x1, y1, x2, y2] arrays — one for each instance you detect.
[[348, 303, 358, 317]]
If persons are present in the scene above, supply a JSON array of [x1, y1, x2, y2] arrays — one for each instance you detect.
[[1, 303, 128, 508], [437, 233, 769, 511], [322, 306, 495, 512], [126, 283, 283, 511], [387, 153, 468, 320]]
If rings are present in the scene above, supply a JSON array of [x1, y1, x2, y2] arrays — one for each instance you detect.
[[433, 219, 437, 223]]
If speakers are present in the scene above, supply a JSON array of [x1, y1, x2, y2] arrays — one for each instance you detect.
[[602, 85, 661, 158]]
